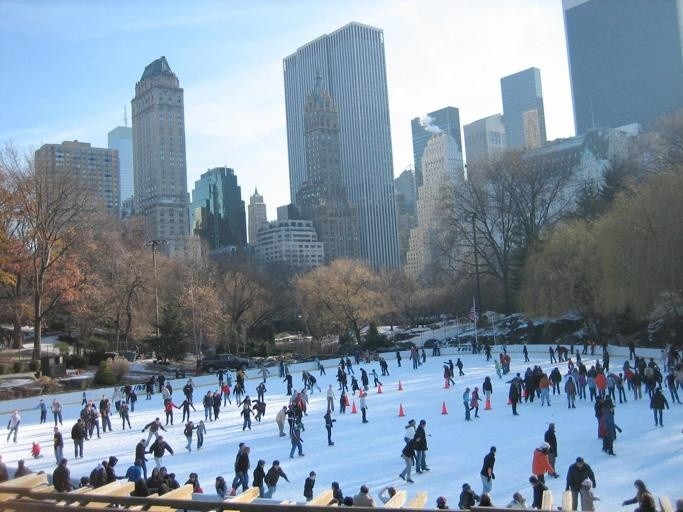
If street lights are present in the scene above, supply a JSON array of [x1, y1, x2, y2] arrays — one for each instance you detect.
[[462, 212, 481, 320], [144, 237, 167, 335]]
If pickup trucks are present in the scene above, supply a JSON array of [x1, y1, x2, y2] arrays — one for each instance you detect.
[[195, 353, 249, 374]]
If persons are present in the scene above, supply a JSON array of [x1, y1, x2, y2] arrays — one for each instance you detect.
[[1, 341, 441, 508], [399, 340, 683, 511]]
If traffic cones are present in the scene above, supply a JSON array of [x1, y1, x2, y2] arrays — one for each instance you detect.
[[342, 394, 349, 407], [443, 378, 452, 390], [357, 387, 365, 398], [396, 380, 404, 391], [440, 400, 450, 415], [482, 387, 529, 411], [350, 401, 358, 414], [397, 403, 405, 417], [375, 383, 384, 394]]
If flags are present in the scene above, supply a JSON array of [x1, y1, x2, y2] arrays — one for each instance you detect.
[[469, 300, 476, 322]]
